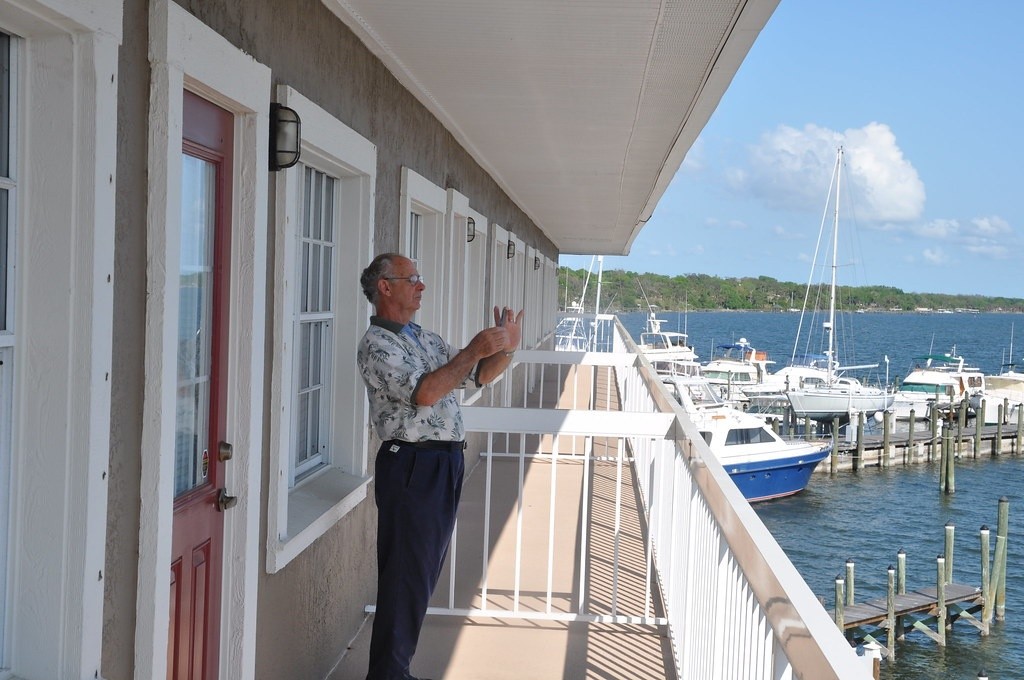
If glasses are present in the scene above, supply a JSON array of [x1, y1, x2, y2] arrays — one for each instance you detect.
[[377, 275, 420, 290]]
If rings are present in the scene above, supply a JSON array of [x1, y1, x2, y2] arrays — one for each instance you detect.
[[509, 320, 515, 322]]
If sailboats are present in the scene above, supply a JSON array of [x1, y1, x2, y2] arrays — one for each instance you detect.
[[571, 144, 1024, 503]]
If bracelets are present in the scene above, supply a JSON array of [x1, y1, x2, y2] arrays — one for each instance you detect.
[[501, 349, 516, 356]]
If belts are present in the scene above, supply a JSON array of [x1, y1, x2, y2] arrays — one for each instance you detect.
[[389, 439, 467, 450]]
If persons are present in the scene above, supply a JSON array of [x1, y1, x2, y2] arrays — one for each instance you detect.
[[357, 252, 526, 680]]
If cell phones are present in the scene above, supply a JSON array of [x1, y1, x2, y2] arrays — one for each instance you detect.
[[499, 309, 507, 327]]
